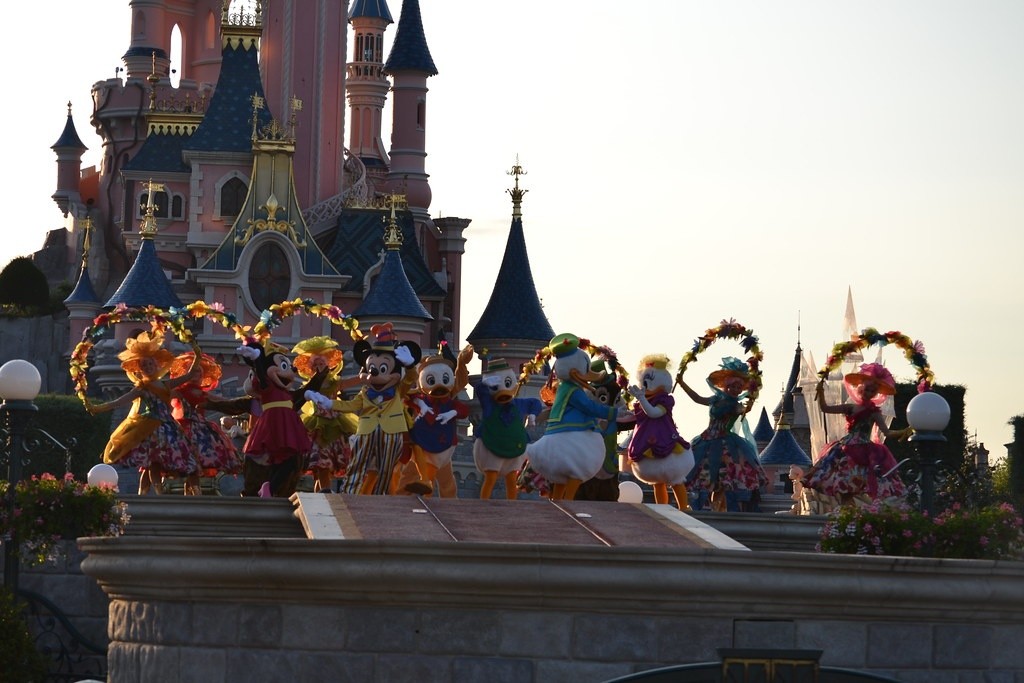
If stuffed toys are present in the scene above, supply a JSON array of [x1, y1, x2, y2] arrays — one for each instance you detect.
[[629, 358, 696, 512], [197, 342, 331, 498], [312, 322, 422, 495], [358, 329, 473, 499], [468, 357, 542, 500], [527, 333, 630, 500]]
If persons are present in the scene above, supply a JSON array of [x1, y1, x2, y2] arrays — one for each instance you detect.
[[138, 351, 241, 496], [88, 330, 199, 496], [787, 465, 808, 501], [515, 376, 559, 499], [676, 358, 750, 493], [800, 363, 913, 509], [291, 336, 361, 491]]
